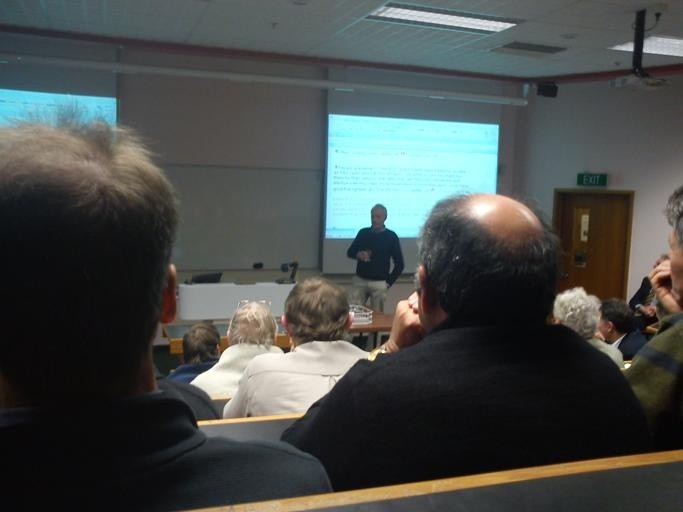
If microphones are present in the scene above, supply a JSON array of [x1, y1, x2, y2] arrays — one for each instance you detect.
[[275, 262, 299, 283]]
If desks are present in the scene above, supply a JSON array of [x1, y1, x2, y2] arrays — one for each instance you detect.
[[161, 313, 400, 359], [178, 280, 298, 321]]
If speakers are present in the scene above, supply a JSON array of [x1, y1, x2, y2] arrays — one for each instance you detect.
[[536, 84, 558, 97]]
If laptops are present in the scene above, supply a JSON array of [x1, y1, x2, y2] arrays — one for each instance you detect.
[[191, 272, 223, 283]]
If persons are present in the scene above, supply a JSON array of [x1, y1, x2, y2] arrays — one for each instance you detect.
[[162, 322, 222, 384], [222, 277, 374, 419], [0, 114, 334, 512], [346, 202, 404, 352], [548, 189, 682, 452], [370, 293, 425, 361], [281, 195, 644, 494], [189, 300, 284, 399]]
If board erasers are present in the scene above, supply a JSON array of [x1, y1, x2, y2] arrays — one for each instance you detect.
[[253, 262, 263, 269]]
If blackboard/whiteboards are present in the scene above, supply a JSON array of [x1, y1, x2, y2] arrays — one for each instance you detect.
[[154, 163, 324, 272]]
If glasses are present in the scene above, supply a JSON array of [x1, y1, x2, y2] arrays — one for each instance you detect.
[[237, 300, 271, 309]]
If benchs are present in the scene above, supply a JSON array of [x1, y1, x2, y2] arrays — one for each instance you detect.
[[197, 412, 306, 442], [189, 446, 683, 512]]
